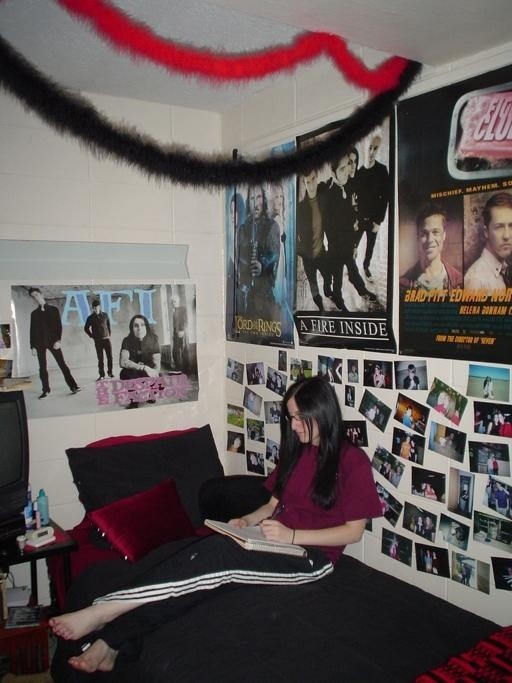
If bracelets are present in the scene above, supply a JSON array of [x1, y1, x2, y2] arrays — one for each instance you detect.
[[291, 529, 295, 545]]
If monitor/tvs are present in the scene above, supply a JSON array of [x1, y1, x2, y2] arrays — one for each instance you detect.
[[0, 389, 30, 523]]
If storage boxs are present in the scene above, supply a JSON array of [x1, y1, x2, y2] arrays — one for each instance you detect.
[[0, 607, 53, 677]]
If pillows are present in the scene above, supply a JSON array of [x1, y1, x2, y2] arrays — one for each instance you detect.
[[175, 475, 273, 537], [86, 480, 196, 562], [65, 424, 225, 512]]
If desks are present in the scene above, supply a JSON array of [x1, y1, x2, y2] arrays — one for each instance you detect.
[[0, 508, 79, 676]]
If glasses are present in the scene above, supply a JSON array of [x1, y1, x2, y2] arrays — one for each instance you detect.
[[285, 413, 305, 422]]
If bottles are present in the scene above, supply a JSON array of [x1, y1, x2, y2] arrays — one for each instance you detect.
[[22, 484, 50, 529]]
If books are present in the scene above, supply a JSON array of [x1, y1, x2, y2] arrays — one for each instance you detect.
[[4, 606, 42, 630], [202, 516, 309, 554]]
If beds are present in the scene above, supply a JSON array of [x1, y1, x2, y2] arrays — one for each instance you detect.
[[62, 520, 512, 683]]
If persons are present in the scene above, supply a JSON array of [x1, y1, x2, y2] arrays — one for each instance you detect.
[[229, 180, 286, 335], [376, 362, 511, 589], [47, 378, 383, 674], [168, 295, 188, 378], [228, 360, 282, 477], [296, 127, 388, 313], [464, 191, 512, 298], [347, 428, 363, 446], [280, 352, 385, 388], [119, 314, 161, 409], [83, 298, 115, 381], [398, 207, 463, 301], [27, 288, 82, 399], [347, 388, 354, 407], [365, 403, 382, 426]]
